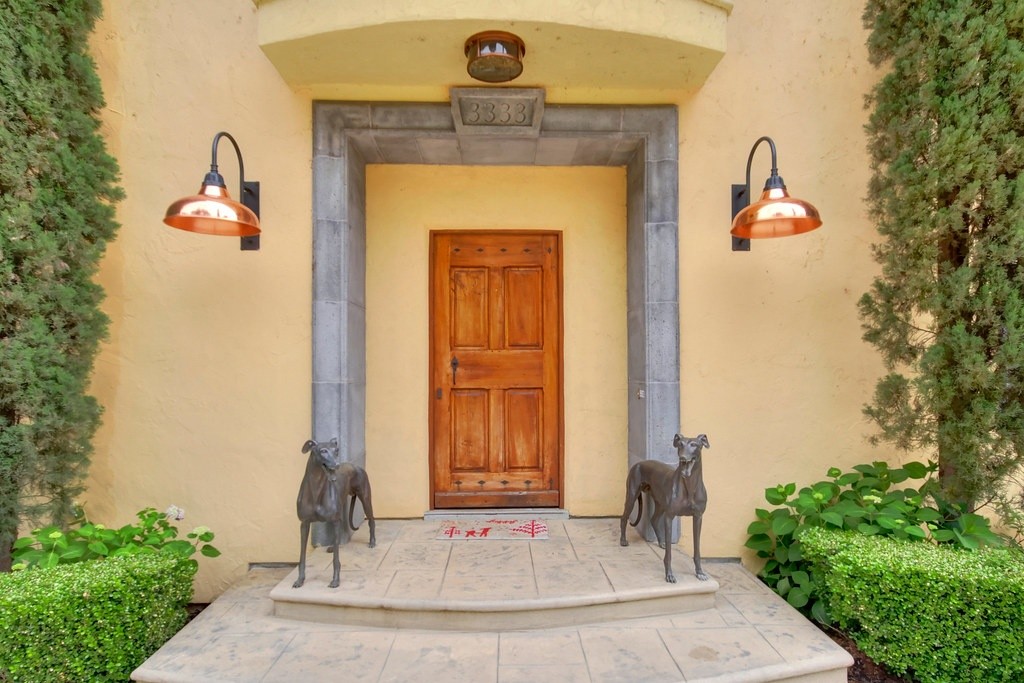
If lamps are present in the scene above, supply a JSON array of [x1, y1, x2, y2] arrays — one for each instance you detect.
[[729, 136, 823, 253], [163, 131, 261, 251]]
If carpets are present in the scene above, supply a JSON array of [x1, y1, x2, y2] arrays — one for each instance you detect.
[[435, 519, 550, 540]]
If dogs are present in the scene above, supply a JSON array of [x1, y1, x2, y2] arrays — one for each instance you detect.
[[619, 433, 711, 584], [292, 438, 376, 589]]
